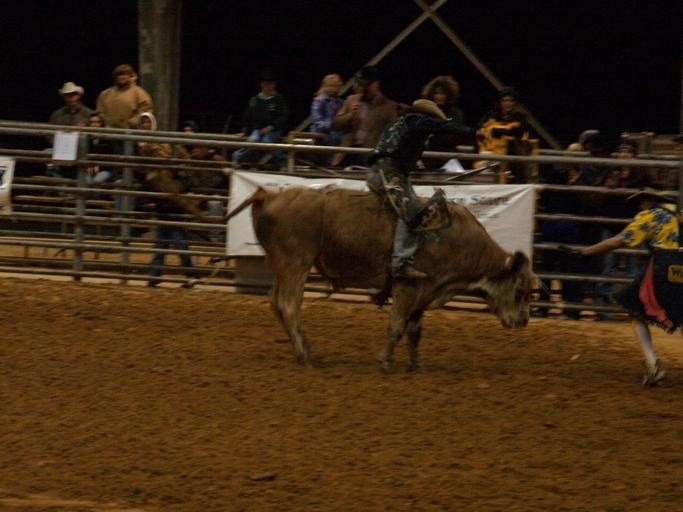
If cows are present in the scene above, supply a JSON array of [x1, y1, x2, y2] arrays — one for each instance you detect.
[[148, 167, 535, 376]]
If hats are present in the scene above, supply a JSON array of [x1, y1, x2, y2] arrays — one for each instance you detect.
[[356, 66, 380, 86], [59, 82, 83, 95], [400, 99, 452, 121], [627, 186, 675, 204]]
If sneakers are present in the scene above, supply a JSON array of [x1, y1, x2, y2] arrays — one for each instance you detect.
[[641, 358, 666, 384]]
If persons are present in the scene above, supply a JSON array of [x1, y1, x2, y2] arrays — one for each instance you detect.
[[230, 73, 681, 321], [367, 102, 489, 279], [40, 63, 157, 234], [567, 186, 681, 386], [135, 114, 226, 287]]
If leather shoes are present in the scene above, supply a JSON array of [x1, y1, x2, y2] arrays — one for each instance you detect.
[[393, 263, 427, 279]]
